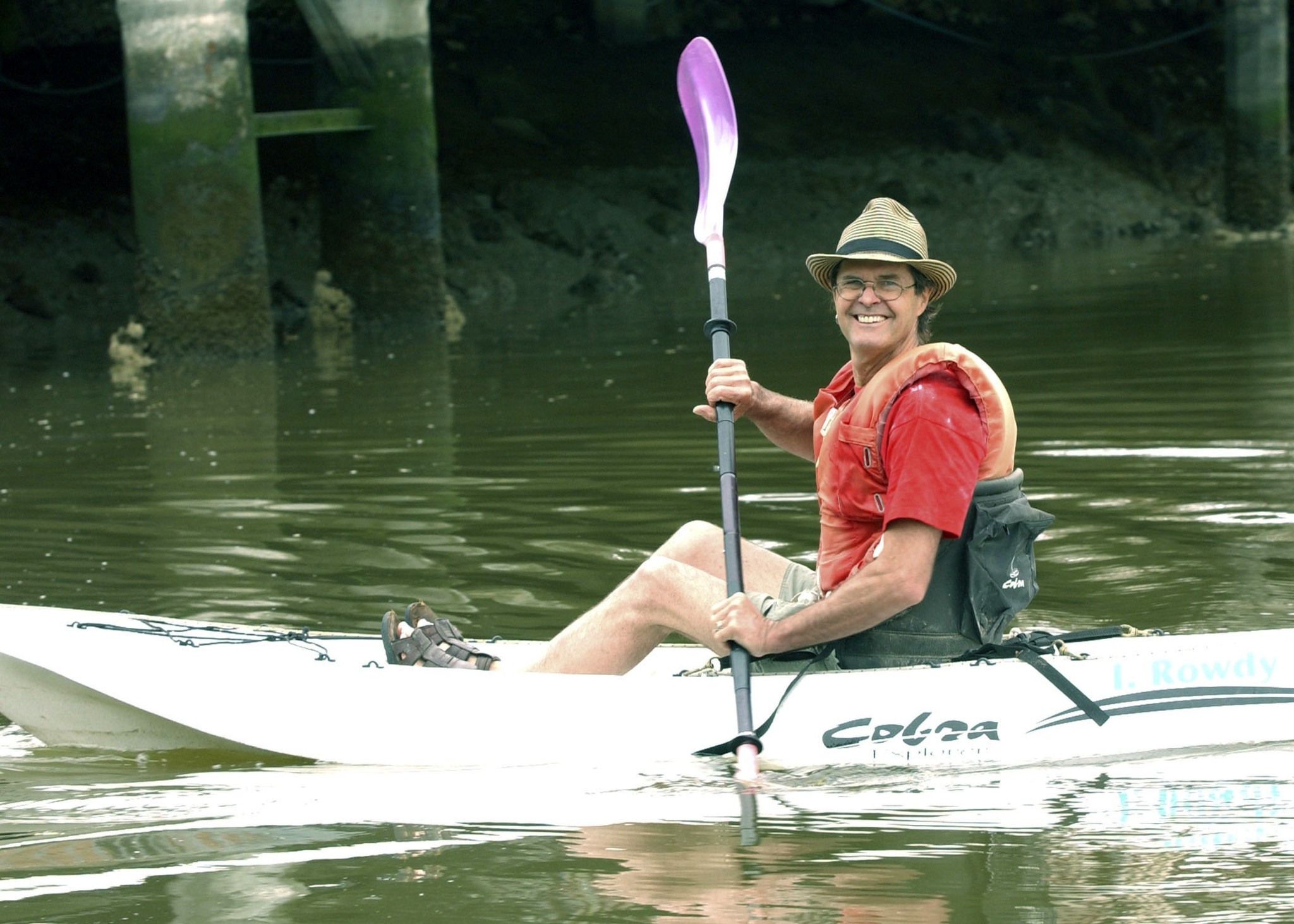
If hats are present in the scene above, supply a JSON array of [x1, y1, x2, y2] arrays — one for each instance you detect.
[[807, 197, 957, 303]]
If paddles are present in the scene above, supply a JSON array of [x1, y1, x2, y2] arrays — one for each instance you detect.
[[677, 36, 766, 847]]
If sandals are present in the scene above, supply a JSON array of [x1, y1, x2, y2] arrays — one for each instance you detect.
[[381, 610, 459, 669], [406, 602, 500, 670]]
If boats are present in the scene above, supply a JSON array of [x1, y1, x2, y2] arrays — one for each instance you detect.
[[0, 602, 1294, 762]]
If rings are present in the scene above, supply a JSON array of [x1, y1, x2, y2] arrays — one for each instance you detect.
[[714, 621, 724, 632]]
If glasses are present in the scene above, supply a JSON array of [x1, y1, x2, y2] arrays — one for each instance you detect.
[[833, 277, 918, 302]]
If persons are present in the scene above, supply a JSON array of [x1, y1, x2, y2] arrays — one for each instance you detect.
[[381, 197, 1019, 675]]
